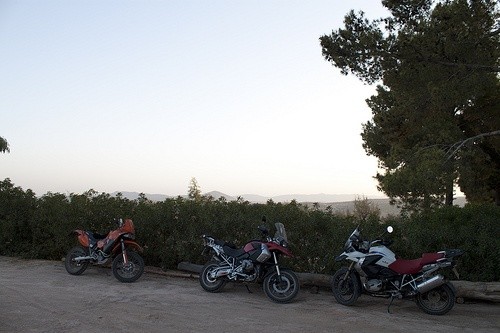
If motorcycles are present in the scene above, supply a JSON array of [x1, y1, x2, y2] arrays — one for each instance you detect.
[[330, 224, 465, 316], [199, 215, 299, 303], [64, 218, 145, 283]]
